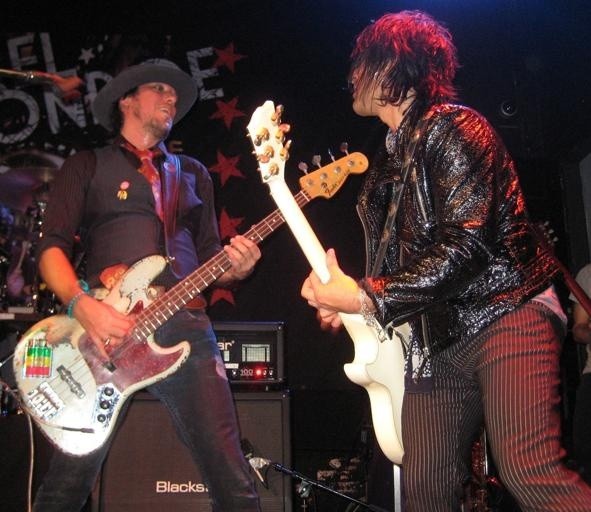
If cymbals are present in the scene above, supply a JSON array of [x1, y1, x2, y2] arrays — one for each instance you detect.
[[0, 165, 62, 216]]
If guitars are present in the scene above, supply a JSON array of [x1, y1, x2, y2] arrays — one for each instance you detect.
[[244, 97, 419, 466], [529, 216, 591, 318], [11, 138, 371, 460]]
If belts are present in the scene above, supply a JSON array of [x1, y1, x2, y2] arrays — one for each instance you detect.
[[184, 296, 207, 311]]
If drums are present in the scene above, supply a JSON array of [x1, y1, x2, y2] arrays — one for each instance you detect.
[[30, 231, 88, 317]]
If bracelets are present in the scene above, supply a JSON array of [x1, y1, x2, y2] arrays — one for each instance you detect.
[[66, 291, 96, 318]]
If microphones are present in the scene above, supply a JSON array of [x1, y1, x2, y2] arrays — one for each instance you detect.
[[239, 440, 271, 489]]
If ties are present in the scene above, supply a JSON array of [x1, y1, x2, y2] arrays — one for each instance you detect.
[[120, 142, 166, 225]]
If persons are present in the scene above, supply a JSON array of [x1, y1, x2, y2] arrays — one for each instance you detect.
[[301, 13, 591, 512], [38, 57, 264, 512], [568, 260, 591, 384]]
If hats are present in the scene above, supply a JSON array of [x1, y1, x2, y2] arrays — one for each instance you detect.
[[92, 57, 198, 137]]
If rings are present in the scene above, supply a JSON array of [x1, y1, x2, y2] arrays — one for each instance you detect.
[[102, 337, 111, 348]]
